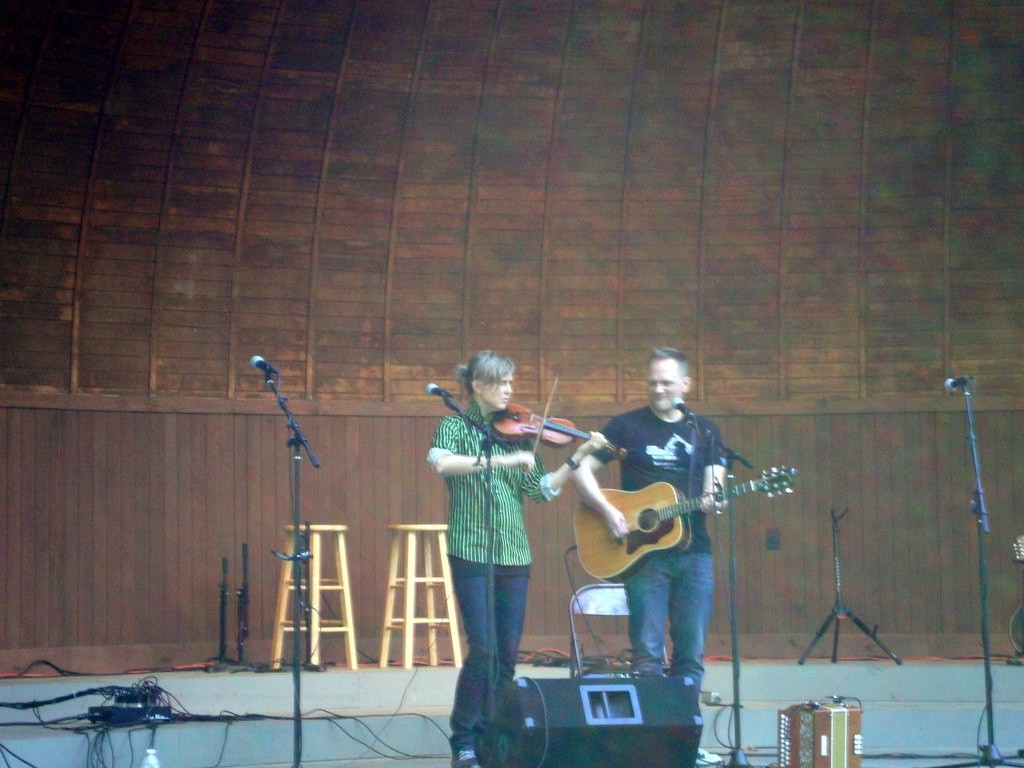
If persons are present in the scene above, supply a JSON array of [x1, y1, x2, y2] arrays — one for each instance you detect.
[[569, 346, 730, 768], [425, 349, 608, 768]]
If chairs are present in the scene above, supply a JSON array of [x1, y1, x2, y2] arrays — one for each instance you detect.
[[569, 583, 667, 678]]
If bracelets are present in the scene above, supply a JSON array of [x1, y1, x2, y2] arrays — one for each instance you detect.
[[565, 455, 579, 470]]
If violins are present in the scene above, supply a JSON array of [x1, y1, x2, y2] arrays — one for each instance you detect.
[[490, 402, 632, 462]]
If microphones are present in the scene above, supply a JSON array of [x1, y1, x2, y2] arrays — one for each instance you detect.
[[673, 397, 696, 419], [250, 355, 280, 374], [426, 383, 454, 398], [944, 376, 975, 391]]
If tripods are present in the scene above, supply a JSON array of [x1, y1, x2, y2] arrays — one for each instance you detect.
[[797, 509, 902, 668], [922, 387, 1024, 768]]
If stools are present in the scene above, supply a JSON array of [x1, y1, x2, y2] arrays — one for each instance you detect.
[[379, 524, 463, 670], [271, 525, 357, 670]]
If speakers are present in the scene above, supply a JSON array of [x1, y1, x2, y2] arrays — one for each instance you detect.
[[475, 676, 703, 768]]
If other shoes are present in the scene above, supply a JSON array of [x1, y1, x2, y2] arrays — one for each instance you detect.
[[695, 748, 722, 766], [451, 748, 481, 768]]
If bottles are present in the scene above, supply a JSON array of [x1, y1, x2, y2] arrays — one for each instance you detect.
[[141, 749, 160, 768]]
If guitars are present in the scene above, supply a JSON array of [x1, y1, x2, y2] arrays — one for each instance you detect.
[[574, 465, 799, 582]]
